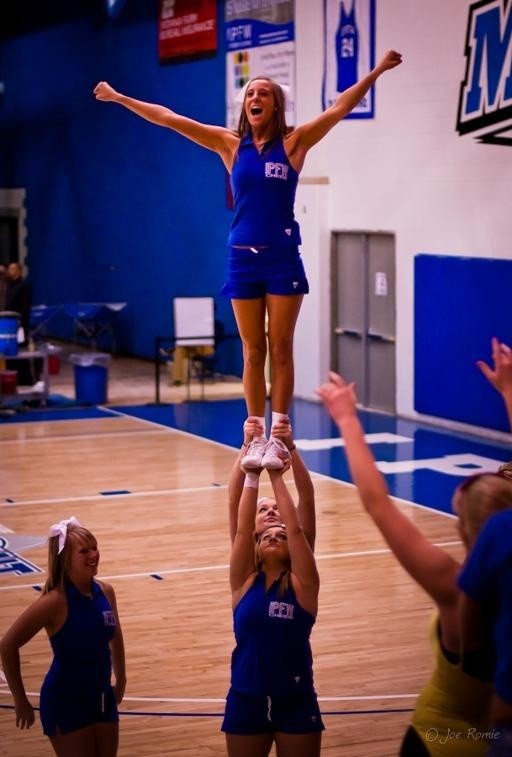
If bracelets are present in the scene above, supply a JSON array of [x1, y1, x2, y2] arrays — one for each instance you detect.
[[241, 441, 251, 449], [289, 444, 297, 452]]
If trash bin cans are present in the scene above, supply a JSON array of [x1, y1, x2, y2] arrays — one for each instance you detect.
[[72, 354, 109, 404]]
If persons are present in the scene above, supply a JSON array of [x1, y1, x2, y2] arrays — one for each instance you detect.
[[94, 50, 405, 471], [221, 451, 326, 757], [224, 416, 317, 555], [455, 507, 512, 757], [312, 336, 512, 757], [0, 517, 127, 757]]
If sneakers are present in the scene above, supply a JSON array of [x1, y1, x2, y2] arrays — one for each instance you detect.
[[241, 437, 284, 470]]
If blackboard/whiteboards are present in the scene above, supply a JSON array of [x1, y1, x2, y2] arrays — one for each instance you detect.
[[174, 298, 215, 346]]
